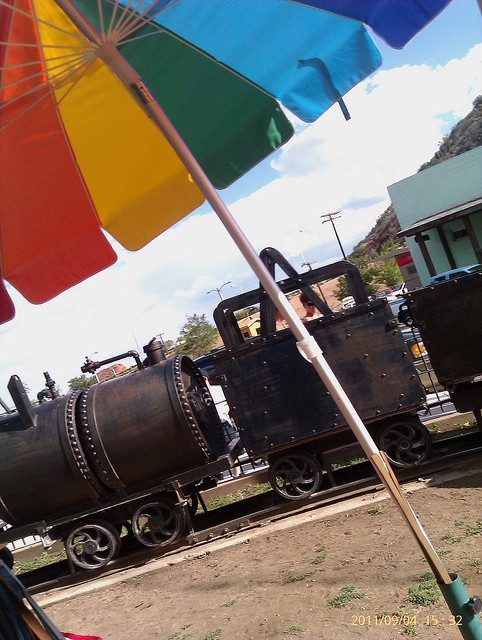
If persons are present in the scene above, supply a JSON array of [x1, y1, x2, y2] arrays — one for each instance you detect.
[[299, 291, 326, 323]]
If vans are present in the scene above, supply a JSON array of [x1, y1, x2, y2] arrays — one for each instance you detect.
[[431, 265, 482, 282]]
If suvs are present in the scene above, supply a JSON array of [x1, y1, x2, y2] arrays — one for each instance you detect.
[[387, 298, 433, 373]]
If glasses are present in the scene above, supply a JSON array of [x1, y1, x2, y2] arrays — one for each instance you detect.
[[304, 303, 314, 307]]
